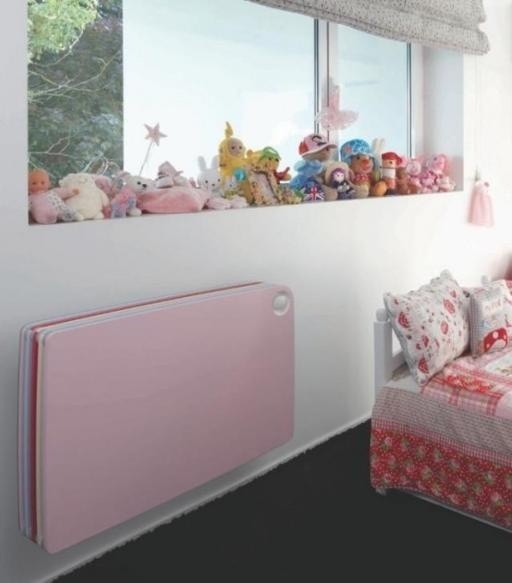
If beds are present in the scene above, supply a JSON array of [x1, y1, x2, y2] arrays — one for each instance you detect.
[[373, 274, 512, 533]]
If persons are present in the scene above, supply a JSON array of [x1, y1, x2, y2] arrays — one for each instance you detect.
[[29, 170, 79, 223]]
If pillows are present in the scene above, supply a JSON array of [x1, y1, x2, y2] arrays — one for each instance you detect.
[[470, 278, 512, 360], [384, 271, 470, 386]]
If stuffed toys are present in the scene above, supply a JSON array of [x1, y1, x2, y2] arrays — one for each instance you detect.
[[107, 171, 151, 219], [146, 133, 453, 212], [59, 173, 109, 220]]
